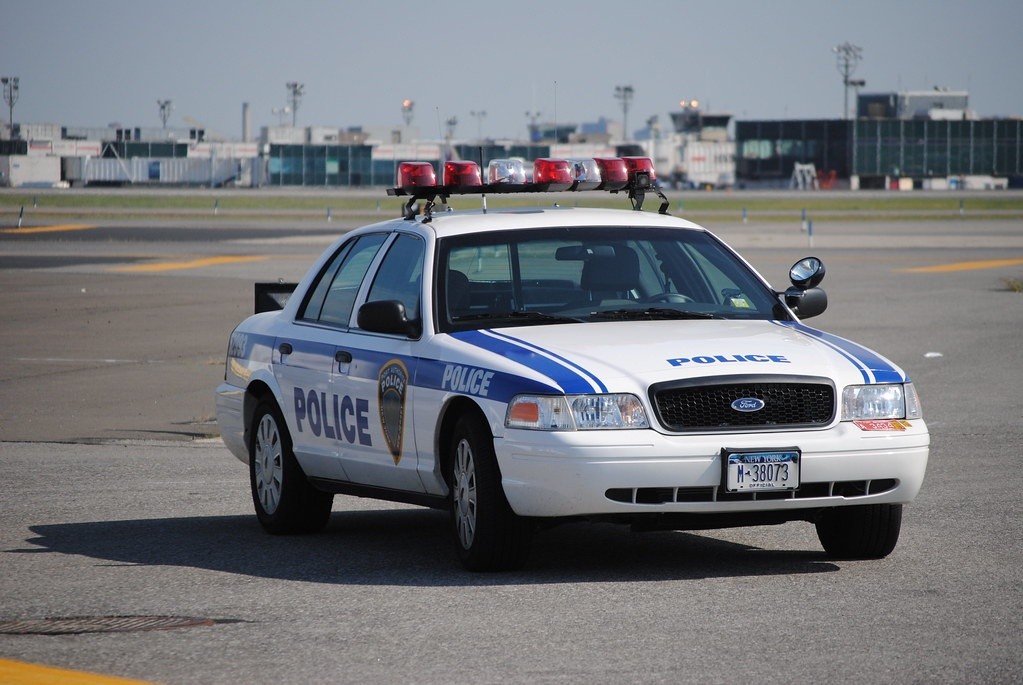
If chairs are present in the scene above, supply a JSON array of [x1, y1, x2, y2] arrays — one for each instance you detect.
[[415, 267, 472, 329], [578, 248, 645, 309]]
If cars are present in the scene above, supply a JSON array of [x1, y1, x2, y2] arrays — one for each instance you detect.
[[211, 154, 931, 561]]
